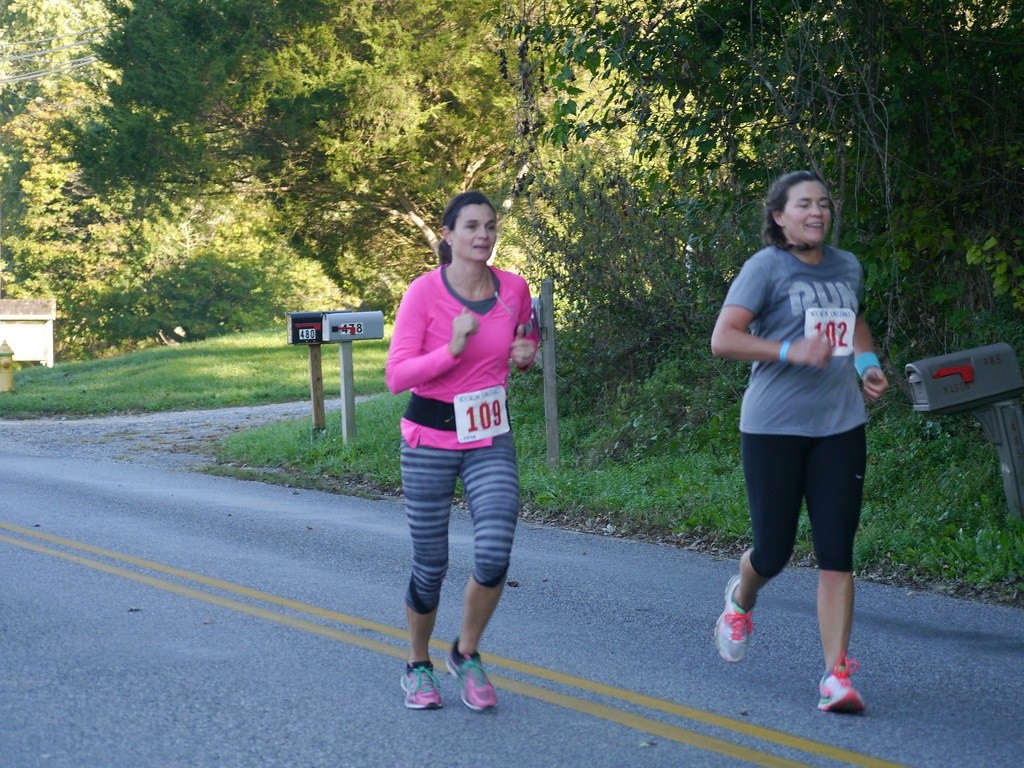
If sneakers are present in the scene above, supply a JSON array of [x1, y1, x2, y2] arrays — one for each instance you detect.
[[817, 648, 866, 711], [400, 655, 442, 710], [446, 637, 496, 713], [714, 575, 758, 663]]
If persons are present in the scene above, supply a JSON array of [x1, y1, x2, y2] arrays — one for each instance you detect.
[[710, 170, 889, 712], [385, 191, 539, 710]]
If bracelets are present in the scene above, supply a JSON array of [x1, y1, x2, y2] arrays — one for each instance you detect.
[[854, 353, 881, 378], [779, 340, 790, 362]]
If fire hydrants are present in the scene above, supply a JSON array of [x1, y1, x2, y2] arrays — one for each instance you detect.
[[0, 340, 15, 392]]
